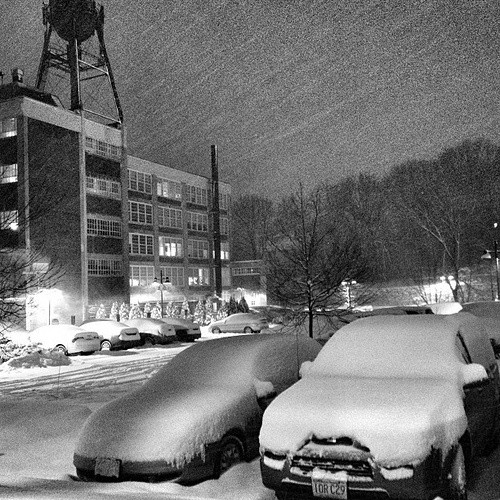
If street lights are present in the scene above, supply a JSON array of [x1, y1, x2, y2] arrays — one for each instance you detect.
[[480, 240, 500, 299], [151, 269, 171, 318]]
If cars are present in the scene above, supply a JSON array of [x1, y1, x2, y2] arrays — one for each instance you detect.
[[207, 312, 269, 334], [0, 321, 33, 363], [71, 332, 323, 488], [79, 321, 142, 352], [124, 319, 177, 346], [258, 299, 500, 500], [159, 317, 201, 342], [30, 323, 101, 357]]
